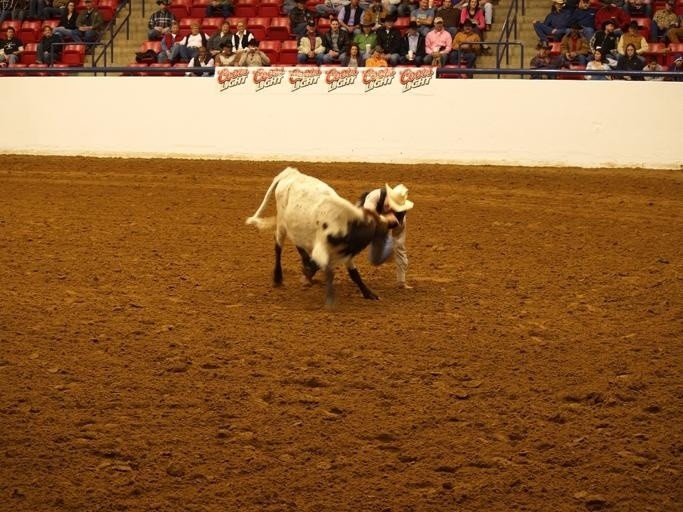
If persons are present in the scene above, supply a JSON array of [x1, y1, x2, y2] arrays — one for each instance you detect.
[[355, 182, 415, 290]]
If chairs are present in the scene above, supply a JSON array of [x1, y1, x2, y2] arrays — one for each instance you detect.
[[0, 0, 682, 80]]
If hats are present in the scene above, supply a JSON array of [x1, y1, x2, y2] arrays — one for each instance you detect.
[[602, 19, 616, 26], [307, 20, 315, 26], [537, 39, 554, 50], [246, 39, 259, 45], [220, 42, 234, 48], [624, 20, 643, 31], [672, 55, 682, 63], [157, 0, 171, 5], [384, 183, 415, 213], [566, 19, 584, 31], [359, 17, 476, 28]]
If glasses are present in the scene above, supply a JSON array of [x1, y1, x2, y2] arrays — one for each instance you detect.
[[667, 3, 674, 6]]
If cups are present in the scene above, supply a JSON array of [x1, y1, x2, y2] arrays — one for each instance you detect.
[[407, 51, 414, 61], [365, 44, 371, 55], [433, 47, 439, 52]]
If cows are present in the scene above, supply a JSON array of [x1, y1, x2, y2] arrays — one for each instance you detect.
[[244, 166, 389, 308]]
